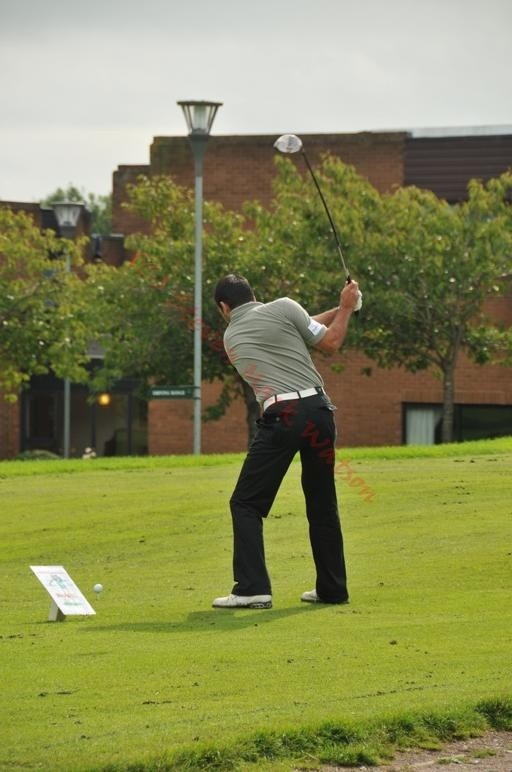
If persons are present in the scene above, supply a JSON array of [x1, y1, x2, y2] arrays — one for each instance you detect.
[[210, 273, 361, 609]]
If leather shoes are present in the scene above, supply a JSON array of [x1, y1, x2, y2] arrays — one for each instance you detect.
[[212, 594, 272, 608], [301, 589, 322, 602]]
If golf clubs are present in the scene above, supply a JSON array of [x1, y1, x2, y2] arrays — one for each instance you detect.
[[272, 133, 360, 320]]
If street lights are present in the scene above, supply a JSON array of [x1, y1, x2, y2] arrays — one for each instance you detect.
[[179, 101, 222, 456], [52, 202, 86, 460]]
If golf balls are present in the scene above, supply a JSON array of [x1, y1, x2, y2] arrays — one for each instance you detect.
[[94, 583, 103, 592]]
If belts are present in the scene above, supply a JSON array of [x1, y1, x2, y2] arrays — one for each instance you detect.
[[263, 387, 323, 412]]
